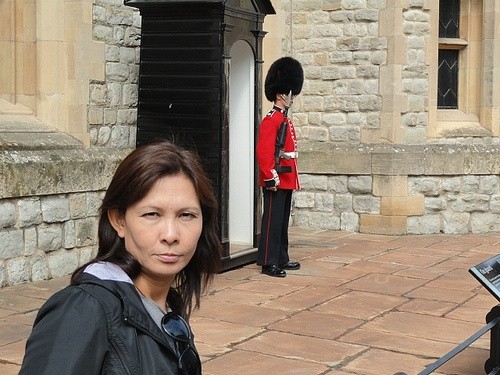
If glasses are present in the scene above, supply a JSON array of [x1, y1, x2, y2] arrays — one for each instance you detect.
[[161, 311, 202, 375]]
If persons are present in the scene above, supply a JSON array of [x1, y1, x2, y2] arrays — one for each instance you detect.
[[256, 84, 300, 277], [17, 139, 227, 375]]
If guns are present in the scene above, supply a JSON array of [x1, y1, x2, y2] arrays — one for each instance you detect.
[[274, 90, 292, 175]]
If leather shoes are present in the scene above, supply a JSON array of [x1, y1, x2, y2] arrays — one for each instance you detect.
[[280, 262, 300, 270], [262, 265, 286, 277]]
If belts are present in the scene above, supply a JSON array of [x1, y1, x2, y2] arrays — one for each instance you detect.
[[282, 152, 298, 158]]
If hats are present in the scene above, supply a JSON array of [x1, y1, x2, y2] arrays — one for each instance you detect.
[[265, 56, 304, 101]]
